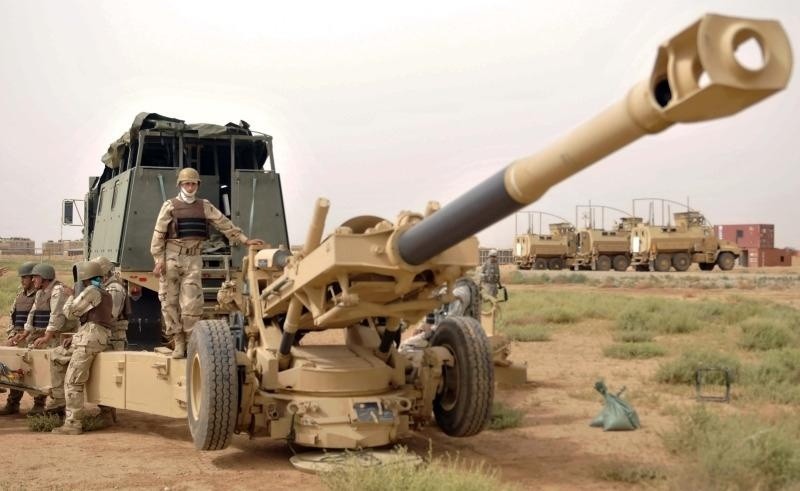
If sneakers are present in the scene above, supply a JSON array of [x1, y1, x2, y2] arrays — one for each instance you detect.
[[52, 422, 82, 434], [172, 337, 185, 359], [95, 410, 116, 426], [0, 405, 45, 415]]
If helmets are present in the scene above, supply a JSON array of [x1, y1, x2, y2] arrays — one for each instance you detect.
[[17, 262, 55, 280], [79, 256, 112, 281], [177, 168, 200, 184]]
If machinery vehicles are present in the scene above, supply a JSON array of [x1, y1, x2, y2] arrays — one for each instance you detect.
[[0, 9, 799, 478], [510, 196, 743, 272]]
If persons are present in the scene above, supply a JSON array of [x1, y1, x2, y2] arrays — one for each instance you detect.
[[13, 264, 76, 415], [149, 168, 266, 358], [0, 262, 49, 415], [478, 249, 500, 298], [398, 272, 482, 352], [53, 259, 109, 436], [91, 256, 129, 425]]
